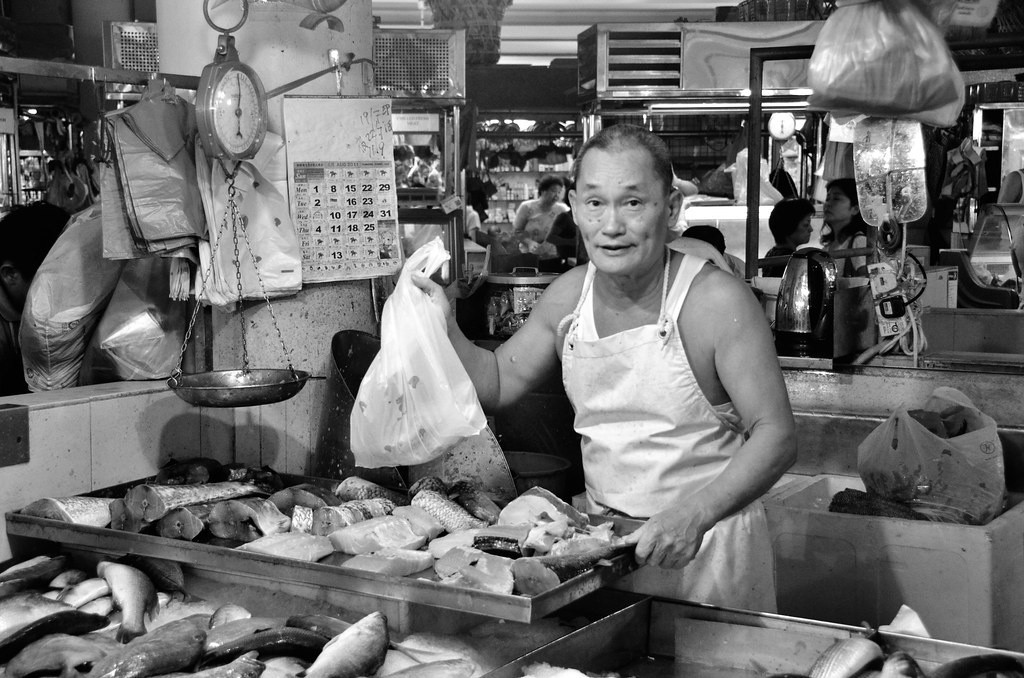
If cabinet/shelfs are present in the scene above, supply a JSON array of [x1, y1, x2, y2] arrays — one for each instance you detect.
[[968, 101, 1022, 204]]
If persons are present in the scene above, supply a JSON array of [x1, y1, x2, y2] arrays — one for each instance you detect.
[[410, 123, 801, 615], [533, 176, 589, 264], [510, 174, 572, 252], [463, 189, 499, 248], [815, 176, 873, 282], [756, 195, 813, 278]]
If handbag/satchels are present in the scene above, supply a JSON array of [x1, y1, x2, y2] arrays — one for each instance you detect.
[[807, 0, 969, 127], [89, 247, 193, 379], [19, 199, 124, 393], [857, 386, 1007, 526], [350, 235, 488, 466]]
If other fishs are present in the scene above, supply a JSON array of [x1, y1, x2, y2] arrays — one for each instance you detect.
[[0, 456, 621, 678], [747, 636, 1023, 678]]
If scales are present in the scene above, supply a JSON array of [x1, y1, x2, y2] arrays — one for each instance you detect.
[[164, 0, 308, 408]]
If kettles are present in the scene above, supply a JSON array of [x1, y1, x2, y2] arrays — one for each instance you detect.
[[772, 245, 839, 360]]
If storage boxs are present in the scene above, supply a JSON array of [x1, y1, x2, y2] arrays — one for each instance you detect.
[[765, 471, 1024, 652], [571, 469, 812, 511]]
